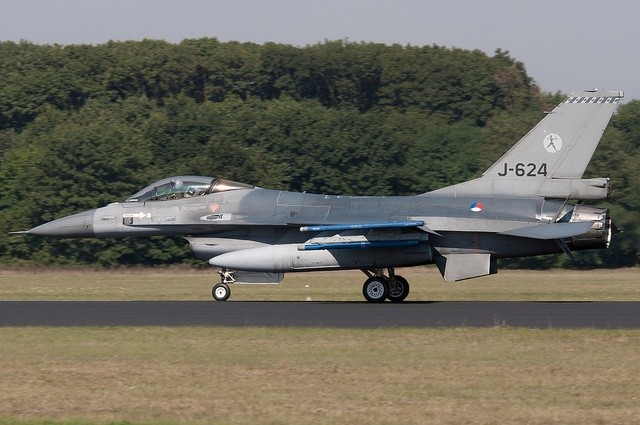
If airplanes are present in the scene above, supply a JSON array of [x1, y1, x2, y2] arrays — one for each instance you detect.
[[8, 89, 625, 302]]
